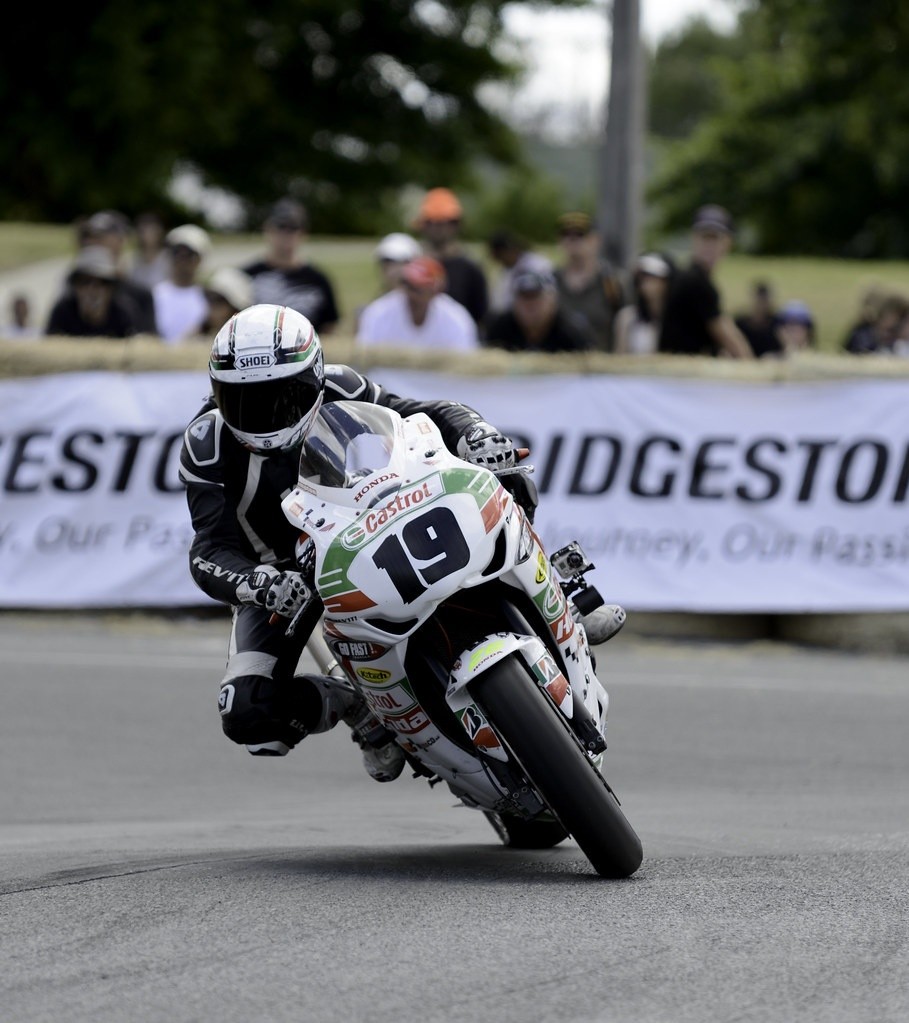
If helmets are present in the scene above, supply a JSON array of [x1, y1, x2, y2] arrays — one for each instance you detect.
[[208, 304, 324, 453]]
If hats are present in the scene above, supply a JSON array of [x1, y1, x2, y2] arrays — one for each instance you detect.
[[86, 213, 123, 236], [77, 247, 117, 275], [404, 257, 446, 292], [165, 224, 209, 258], [559, 213, 591, 236], [267, 200, 303, 224], [695, 207, 734, 234], [771, 307, 813, 331], [636, 250, 673, 280], [378, 232, 419, 262]]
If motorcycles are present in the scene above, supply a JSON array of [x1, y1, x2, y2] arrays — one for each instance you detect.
[[264, 399, 645, 883]]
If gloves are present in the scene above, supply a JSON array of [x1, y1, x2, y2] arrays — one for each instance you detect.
[[456, 420, 514, 472], [238, 563, 314, 617]]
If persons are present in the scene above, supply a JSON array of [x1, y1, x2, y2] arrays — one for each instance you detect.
[[39, 185, 908, 360], [178, 304, 626, 781]]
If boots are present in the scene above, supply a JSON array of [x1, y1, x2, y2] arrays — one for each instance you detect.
[[560, 586, 628, 644], [297, 674, 404, 782]]
